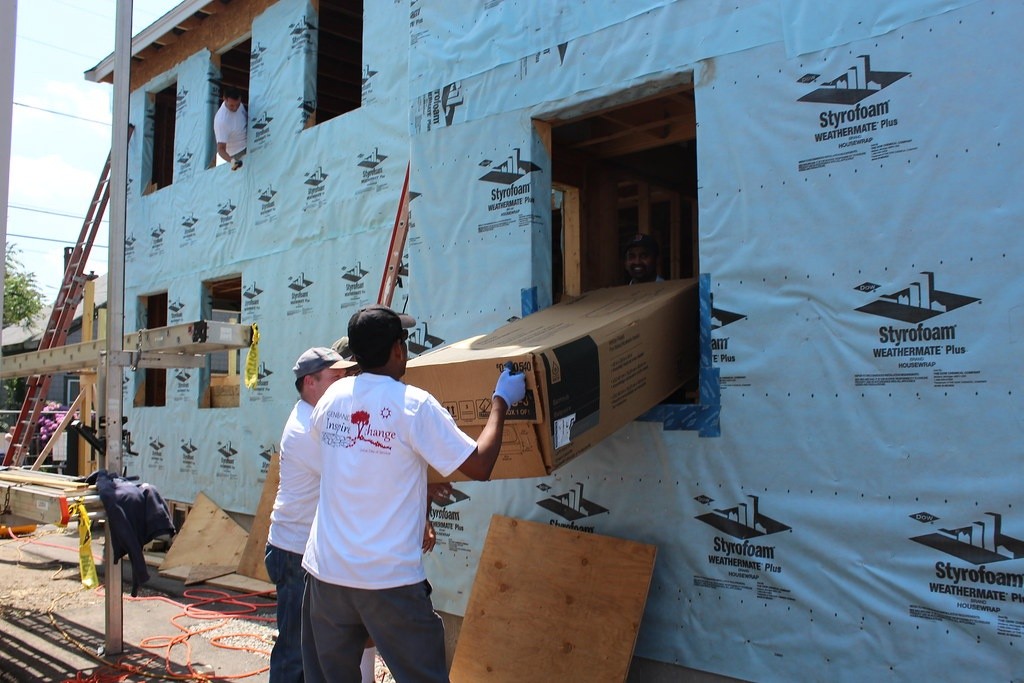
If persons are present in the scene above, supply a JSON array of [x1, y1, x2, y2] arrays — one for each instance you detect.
[[301, 305, 525, 683], [624, 234, 666, 286], [328, 336, 436, 683], [265, 347, 360, 683], [5, 425, 16, 451], [213, 87, 247, 171]]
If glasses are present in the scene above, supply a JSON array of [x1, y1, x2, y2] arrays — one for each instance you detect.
[[401, 328, 409, 342]]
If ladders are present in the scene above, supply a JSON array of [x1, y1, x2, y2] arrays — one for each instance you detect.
[[1, 124, 139, 470], [374, 161, 412, 309]]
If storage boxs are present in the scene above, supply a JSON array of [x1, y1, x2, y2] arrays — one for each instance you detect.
[[399, 279, 699, 485]]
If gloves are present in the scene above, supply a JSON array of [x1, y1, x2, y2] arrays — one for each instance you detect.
[[492, 361, 527, 415], [231, 159, 238, 170]]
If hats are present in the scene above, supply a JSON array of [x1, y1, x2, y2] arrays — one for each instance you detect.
[[292, 347, 358, 378], [348, 304, 416, 351], [331, 336, 353, 361]]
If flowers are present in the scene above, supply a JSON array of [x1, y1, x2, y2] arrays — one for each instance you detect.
[[37, 399, 95, 441]]
